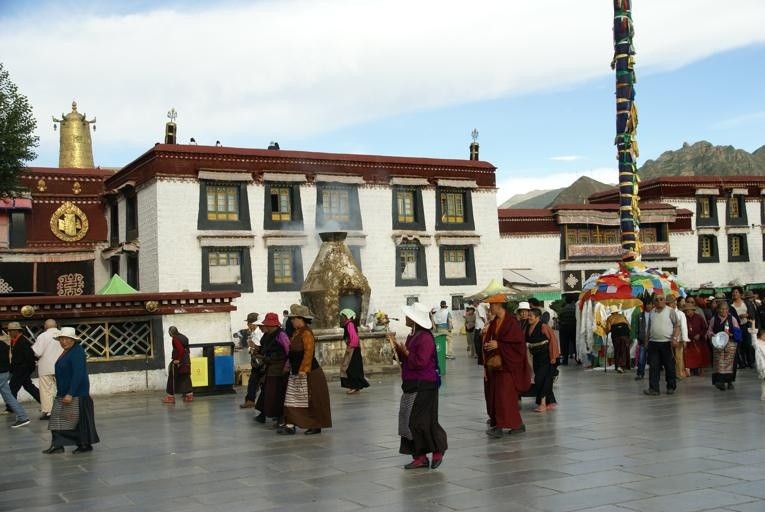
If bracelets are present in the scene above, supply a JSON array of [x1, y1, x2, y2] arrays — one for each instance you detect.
[[403, 350, 407, 352]]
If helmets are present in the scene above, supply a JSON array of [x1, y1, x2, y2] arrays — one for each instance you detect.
[[744, 291, 755, 297]]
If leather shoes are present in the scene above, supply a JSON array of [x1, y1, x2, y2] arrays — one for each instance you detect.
[[431, 449, 445, 469], [41, 443, 92, 453], [274, 415, 322, 435], [405, 457, 429, 469]]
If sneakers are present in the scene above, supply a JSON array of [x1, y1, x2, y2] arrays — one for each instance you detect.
[[485, 427, 503, 438], [533, 404, 547, 412], [715, 381, 734, 391], [617, 366, 625, 373], [183, 392, 193, 402], [644, 387, 676, 396], [546, 403, 557, 410], [346, 388, 358, 394], [684, 367, 691, 376], [635, 374, 643, 379], [239, 400, 267, 425], [508, 424, 525, 434], [161, 394, 174, 403], [1, 409, 52, 429]]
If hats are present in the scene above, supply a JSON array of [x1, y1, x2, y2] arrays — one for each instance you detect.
[[285, 304, 309, 317], [681, 303, 696, 312], [665, 294, 677, 303], [712, 292, 729, 301], [712, 331, 729, 349], [242, 312, 281, 327], [52, 327, 82, 345], [401, 303, 433, 330], [608, 305, 621, 314], [481, 295, 510, 303], [7, 322, 23, 331], [513, 302, 531, 314]]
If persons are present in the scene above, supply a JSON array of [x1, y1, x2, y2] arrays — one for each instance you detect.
[[30, 319, 61, 420], [428, 307, 437, 321], [233, 304, 332, 435], [41, 326, 100, 453], [386, 302, 447, 469], [605, 286, 760, 395], [339, 308, 369, 394], [161, 326, 194, 403], [465, 293, 576, 436], [0, 331, 30, 429], [0, 322, 40, 415], [434, 301, 455, 358]]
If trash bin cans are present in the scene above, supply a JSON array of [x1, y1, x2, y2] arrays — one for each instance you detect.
[[434, 334, 447, 376], [187, 342, 236, 397]]
